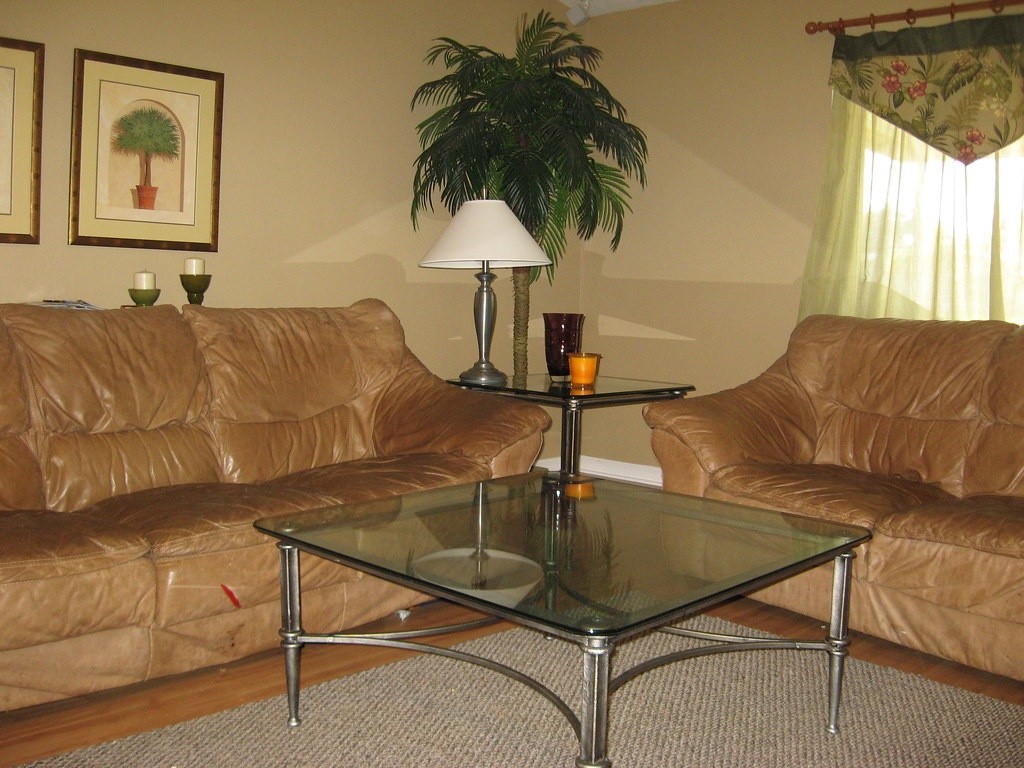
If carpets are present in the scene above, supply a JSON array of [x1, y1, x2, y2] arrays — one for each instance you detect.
[[19, 611, 1024, 768]]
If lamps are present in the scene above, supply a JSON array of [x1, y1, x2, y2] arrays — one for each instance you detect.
[[419, 199, 554, 385]]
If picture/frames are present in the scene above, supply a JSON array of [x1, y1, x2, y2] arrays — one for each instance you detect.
[[68, 45, 225, 253], [0, 37, 46, 245]]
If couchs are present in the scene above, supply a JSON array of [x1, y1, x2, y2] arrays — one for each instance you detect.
[[0, 295, 553, 768], [643, 314, 1024, 683]]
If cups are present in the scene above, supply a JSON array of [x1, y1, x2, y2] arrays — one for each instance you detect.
[[542, 313, 583, 382], [539, 472, 576, 530], [567, 353, 600, 390], [565, 475, 595, 501]]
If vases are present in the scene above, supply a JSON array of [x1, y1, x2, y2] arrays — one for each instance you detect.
[[543, 311, 586, 383]]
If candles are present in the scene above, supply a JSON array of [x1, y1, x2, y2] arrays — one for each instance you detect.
[[133, 268, 156, 290], [183, 256, 205, 276]]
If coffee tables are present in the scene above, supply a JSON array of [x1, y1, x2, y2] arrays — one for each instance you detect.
[[252, 470, 875, 758], [447, 371, 695, 577]]
